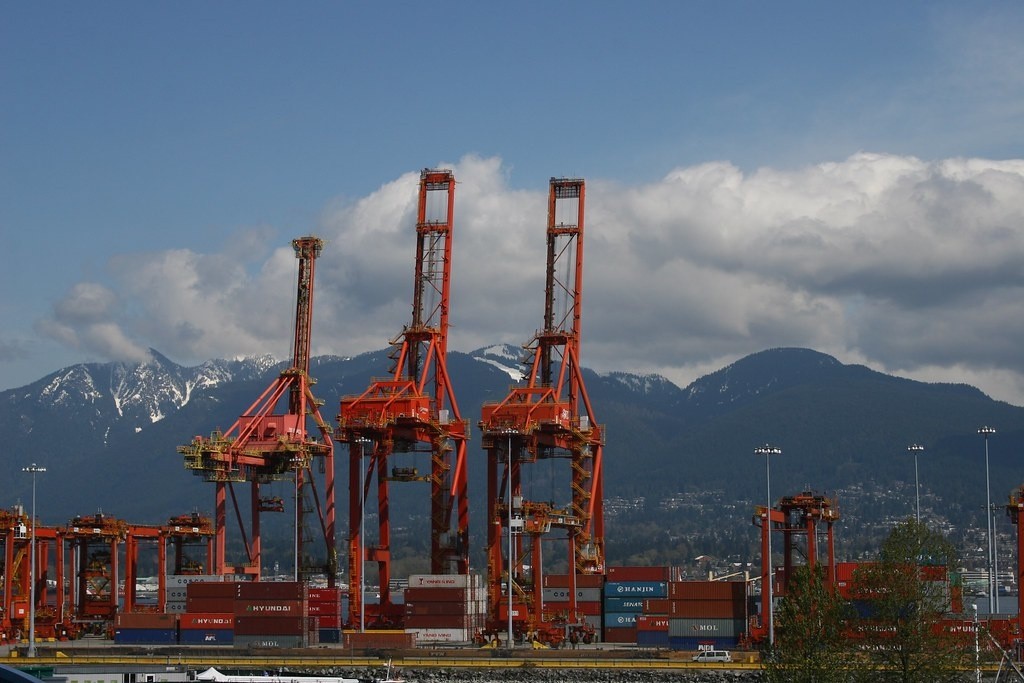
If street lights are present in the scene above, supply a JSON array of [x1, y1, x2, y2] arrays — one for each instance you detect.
[[23, 462, 47, 658], [754, 442, 785, 656], [977, 425, 994, 617], [980, 502, 1003, 615], [907, 443, 928, 651]]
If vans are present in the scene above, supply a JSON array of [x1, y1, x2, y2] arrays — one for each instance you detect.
[[692, 651, 732, 663]]
